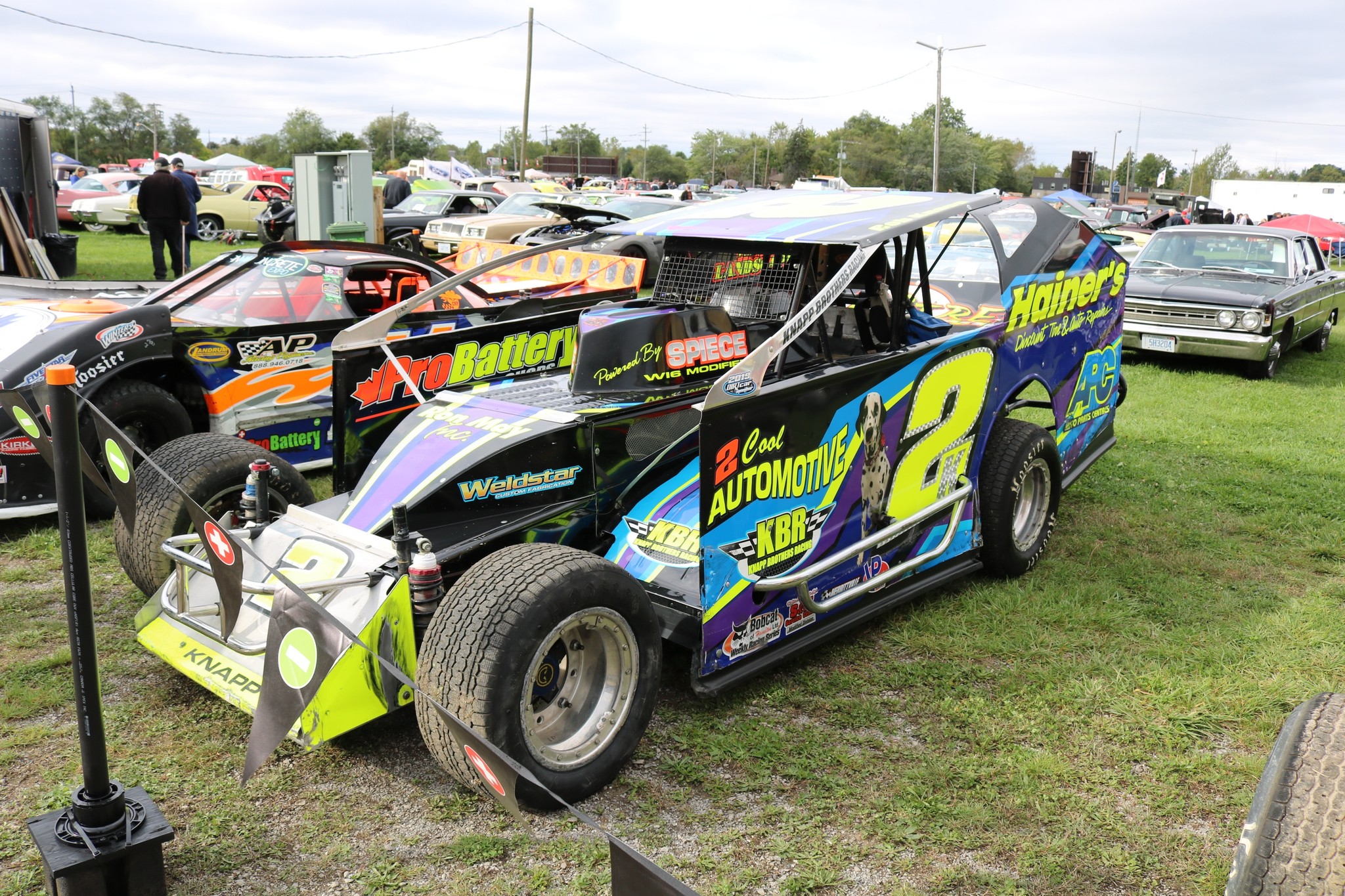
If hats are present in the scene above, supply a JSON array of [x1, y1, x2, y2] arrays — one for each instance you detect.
[[154, 157, 171, 167], [171, 158, 183, 167], [132, 167, 140, 171]]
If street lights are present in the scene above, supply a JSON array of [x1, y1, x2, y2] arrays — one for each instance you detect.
[[1185, 163, 1193, 195], [707, 128, 717, 186], [1109, 130, 1123, 199], [135, 122, 157, 151], [916, 41, 986, 193], [829, 158, 842, 177]]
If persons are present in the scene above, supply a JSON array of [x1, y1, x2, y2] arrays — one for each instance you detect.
[[70, 167, 87, 185], [505, 173, 748, 203], [168, 158, 202, 272], [382, 169, 413, 209], [137, 158, 190, 279], [1137, 189, 1340, 234], [1050, 201, 1102, 213], [127, 166, 142, 191]]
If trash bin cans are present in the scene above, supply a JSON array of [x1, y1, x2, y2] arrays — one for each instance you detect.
[[43, 235, 78, 276], [325, 220, 368, 243]]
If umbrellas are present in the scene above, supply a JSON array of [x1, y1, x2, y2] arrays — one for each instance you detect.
[[1042, 189, 1097, 208]]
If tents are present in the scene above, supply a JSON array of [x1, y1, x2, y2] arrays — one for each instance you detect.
[[51, 151, 268, 183], [1245, 214, 1345, 267]]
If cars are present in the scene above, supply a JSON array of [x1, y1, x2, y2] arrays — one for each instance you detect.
[[867, 196, 1345, 381], [115, 189, 1130, 844], [0, 240, 646, 520], [57, 164, 848, 290]]
